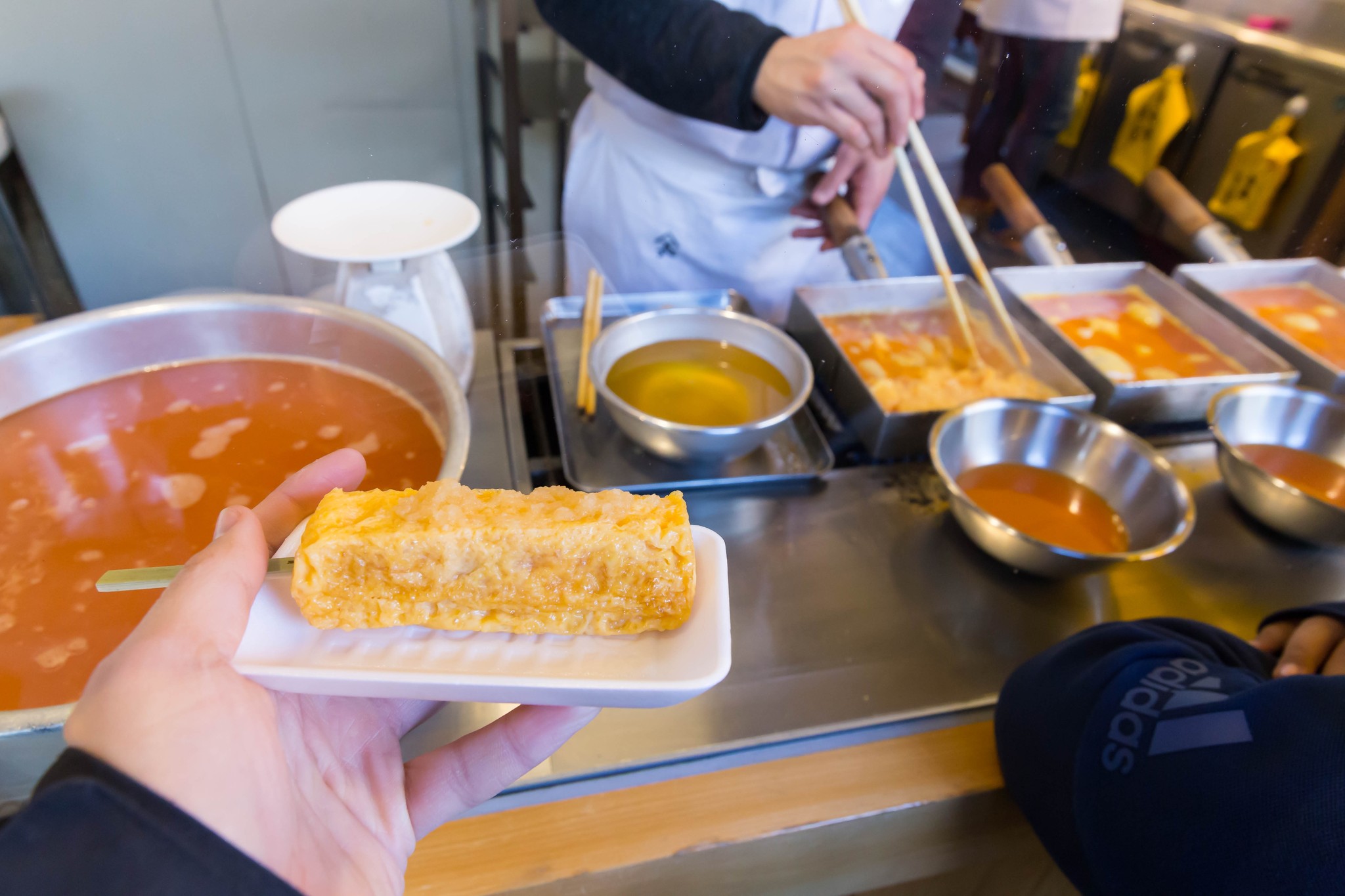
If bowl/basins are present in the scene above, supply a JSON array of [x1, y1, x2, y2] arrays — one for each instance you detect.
[[589, 306, 815, 460], [926, 396, 1198, 571], [0, 292, 470, 734], [1204, 386, 1344, 540]]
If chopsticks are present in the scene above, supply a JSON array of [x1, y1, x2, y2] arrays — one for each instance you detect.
[[577, 267, 606, 420], [841, 0, 1036, 370]]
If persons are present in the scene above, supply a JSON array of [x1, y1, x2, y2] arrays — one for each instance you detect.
[[0, 447, 598, 896], [955, 1, 1126, 260], [990, 600, 1344, 895], [524, 0, 925, 333]]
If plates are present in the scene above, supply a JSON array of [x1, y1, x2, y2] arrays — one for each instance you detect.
[[271, 174, 481, 261], [227, 516, 732, 710]]
